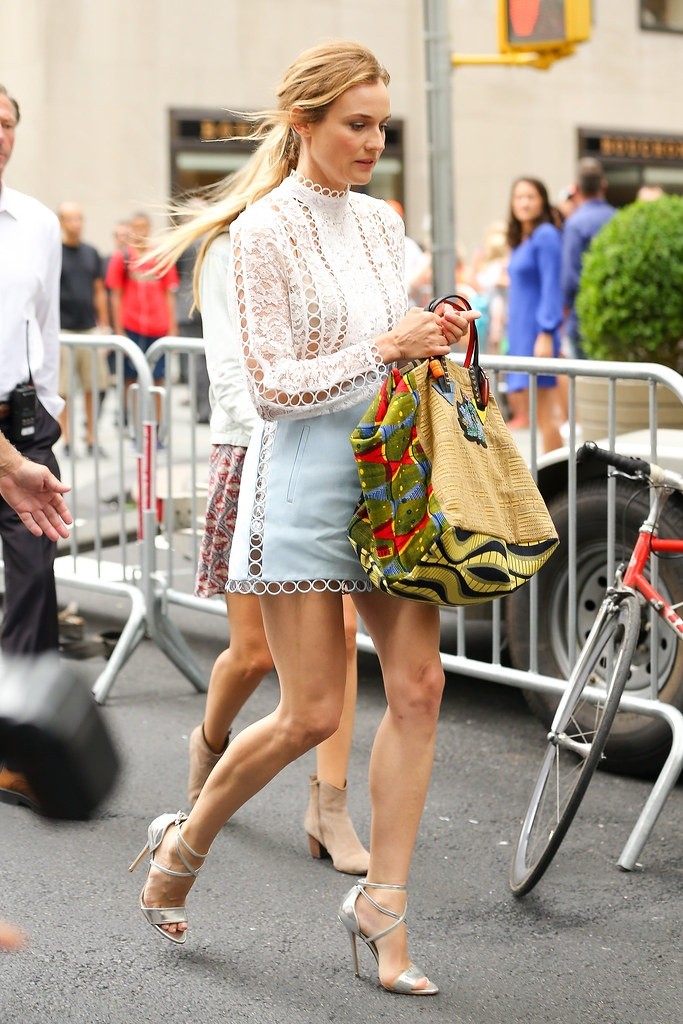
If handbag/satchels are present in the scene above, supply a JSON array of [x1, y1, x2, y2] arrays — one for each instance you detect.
[[345, 295, 560, 607]]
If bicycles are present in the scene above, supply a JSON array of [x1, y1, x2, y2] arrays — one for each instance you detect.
[[506, 437, 682, 898]]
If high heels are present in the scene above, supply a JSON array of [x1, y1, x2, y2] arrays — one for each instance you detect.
[[337, 878, 440, 995], [188, 720, 232, 811], [128, 809, 211, 944], [304, 774, 370, 874]]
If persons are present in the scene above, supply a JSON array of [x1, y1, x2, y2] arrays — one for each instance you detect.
[[125, 41, 482, 997], [381, 173, 675, 428], [107, 215, 179, 454], [0, 411, 75, 541], [502, 178, 562, 453], [0, 83, 63, 818], [58, 208, 114, 455], [559, 159, 618, 365], [189, 207, 373, 876]]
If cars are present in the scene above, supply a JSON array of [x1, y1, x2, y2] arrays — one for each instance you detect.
[[482, 428, 682, 783]]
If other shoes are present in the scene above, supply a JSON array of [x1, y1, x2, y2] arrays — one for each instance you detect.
[[64, 445, 79, 460], [87, 445, 108, 460]]
